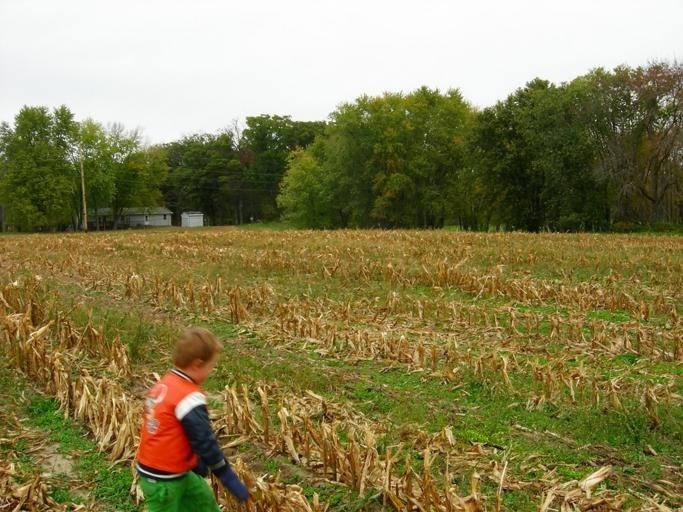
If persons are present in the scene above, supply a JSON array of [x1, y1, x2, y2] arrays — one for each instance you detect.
[[133, 324, 254, 512]]
